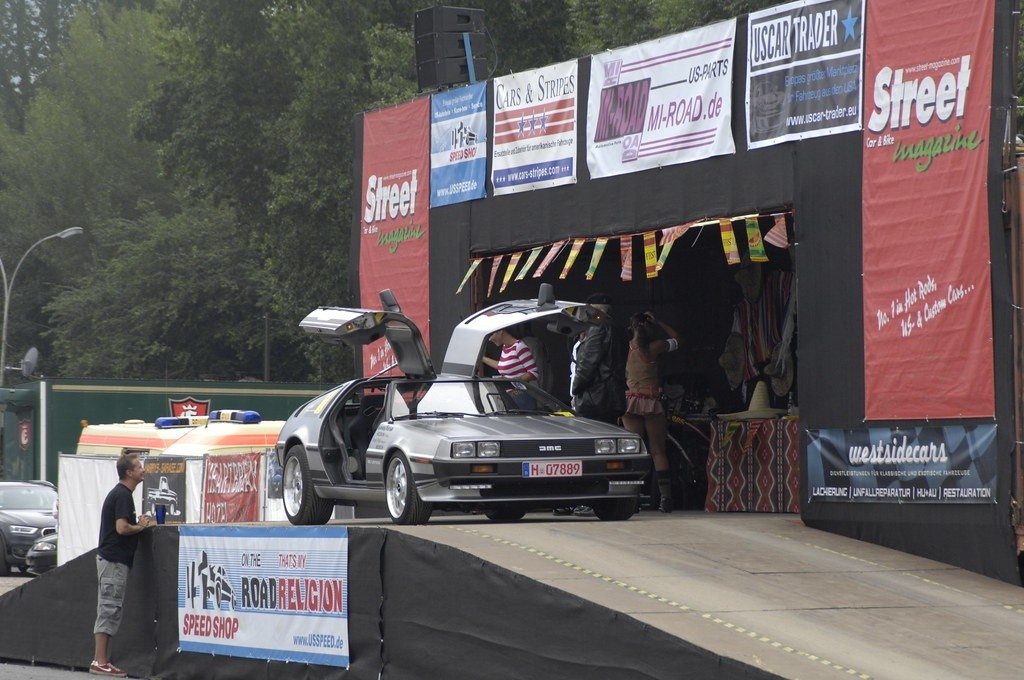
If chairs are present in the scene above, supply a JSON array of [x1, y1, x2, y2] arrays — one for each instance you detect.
[[346, 395, 386, 478]]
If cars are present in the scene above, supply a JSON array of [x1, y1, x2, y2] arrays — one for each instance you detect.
[[274, 282, 656, 525], [0, 481, 61, 574]]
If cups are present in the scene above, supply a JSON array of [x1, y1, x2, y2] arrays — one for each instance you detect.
[[155, 504, 167, 524]]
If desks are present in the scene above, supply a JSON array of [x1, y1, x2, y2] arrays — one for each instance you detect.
[[705, 418, 799, 513]]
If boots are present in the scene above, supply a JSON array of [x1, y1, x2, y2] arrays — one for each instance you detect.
[[655, 471, 675, 513]]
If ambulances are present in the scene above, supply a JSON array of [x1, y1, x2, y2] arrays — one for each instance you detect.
[[159, 408, 336, 521], [76, 409, 209, 457]]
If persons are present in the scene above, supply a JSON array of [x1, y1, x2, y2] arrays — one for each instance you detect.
[[482, 328, 539, 383], [572, 292, 627, 425], [621, 309, 685, 514], [514, 322, 554, 395], [87, 452, 149, 677], [569, 329, 587, 412]]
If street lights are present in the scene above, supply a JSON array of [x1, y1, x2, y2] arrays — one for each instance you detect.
[[0, 227, 85, 385]]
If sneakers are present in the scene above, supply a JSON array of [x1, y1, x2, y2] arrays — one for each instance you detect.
[[88, 660, 127, 677]]
